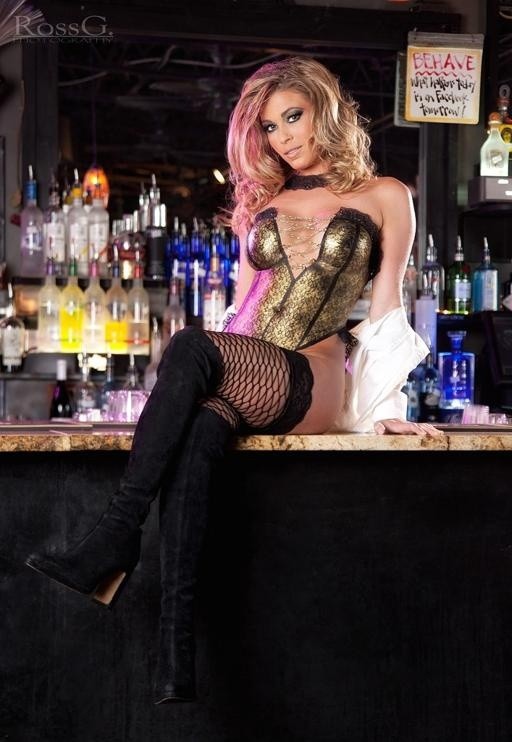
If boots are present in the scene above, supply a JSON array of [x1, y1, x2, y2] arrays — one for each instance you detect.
[[24, 328, 224, 606], [147, 405, 237, 718]]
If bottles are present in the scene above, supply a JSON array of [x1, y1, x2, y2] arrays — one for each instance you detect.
[[48, 357, 75, 422], [121, 352, 141, 392], [404, 330, 477, 424], [486, 84, 512, 153], [477, 112, 509, 178], [170, 214, 239, 308], [163, 276, 185, 348], [95, 354, 118, 405], [1, 302, 28, 374], [72, 351, 101, 417], [196, 249, 227, 336], [1, 179, 165, 360], [367, 228, 500, 318]]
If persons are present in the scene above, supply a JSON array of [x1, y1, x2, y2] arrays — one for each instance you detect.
[[28, 55, 444, 701]]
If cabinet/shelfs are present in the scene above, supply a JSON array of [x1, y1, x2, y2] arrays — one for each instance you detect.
[[439, 199, 511, 334], [9, 264, 172, 377]]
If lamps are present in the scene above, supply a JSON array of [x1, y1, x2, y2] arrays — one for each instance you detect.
[[81, 92, 111, 208]]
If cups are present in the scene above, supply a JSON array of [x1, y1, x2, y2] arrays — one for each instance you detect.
[[103, 387, 151, 426], [460, 399, 507, 428]]
